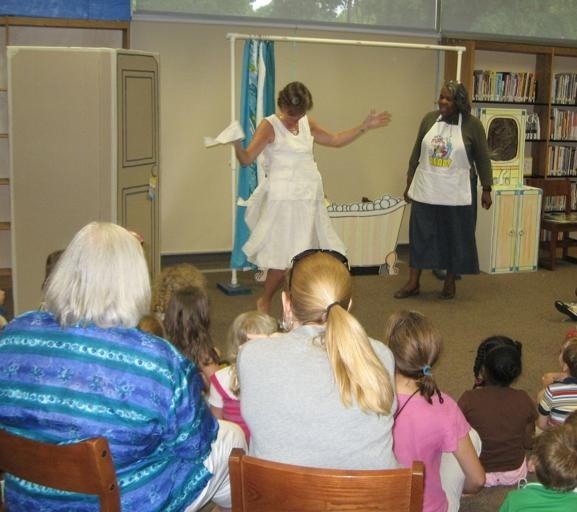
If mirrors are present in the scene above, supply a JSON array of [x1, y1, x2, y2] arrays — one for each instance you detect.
[[486, 117, 520, 164]]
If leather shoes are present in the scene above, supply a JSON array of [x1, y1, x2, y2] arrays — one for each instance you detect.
[[439, 280, 456, 299], [394, 284, 421, 298]]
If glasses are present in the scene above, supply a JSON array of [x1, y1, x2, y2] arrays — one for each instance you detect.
[[288, 249, 351, 302]]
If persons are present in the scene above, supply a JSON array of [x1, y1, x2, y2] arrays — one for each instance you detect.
[[231, 80, 392, 314], [530, 340, 577, 436], [394, 80, 493, 299], [207, 312, 280, 443], [500, 424, 577, 511], [457, 336, 538, 487], [152, 264, 207, 333], [384, 307, 486, 511], [234, 250, 399, 469], [0, 221, 248, 512], [163, 287, 222, 383]]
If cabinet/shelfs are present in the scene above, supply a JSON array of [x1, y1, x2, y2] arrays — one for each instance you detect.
[[478, 184, 541, 275], [444, 38, 576, 245]]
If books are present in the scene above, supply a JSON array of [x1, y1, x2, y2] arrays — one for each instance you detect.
[[547, 145, 577, 176], [548, 108, 576, 141], [542, 182, 577, 213], [474, 69, 537, 102], [541, 215, 577, 241], [526, 113, 541, 139], [552, 72, 577, 105]]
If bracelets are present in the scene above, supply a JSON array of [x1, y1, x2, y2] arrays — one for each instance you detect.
[[482, 188, 491, 192]]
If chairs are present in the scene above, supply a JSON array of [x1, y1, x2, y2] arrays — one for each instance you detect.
[[529, 178, 576, 271], [0, 429, 121, 512], [227, 447, 425, 512]]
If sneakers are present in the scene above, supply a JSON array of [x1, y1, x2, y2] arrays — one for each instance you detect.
[[554, 299, 577, 322]]
